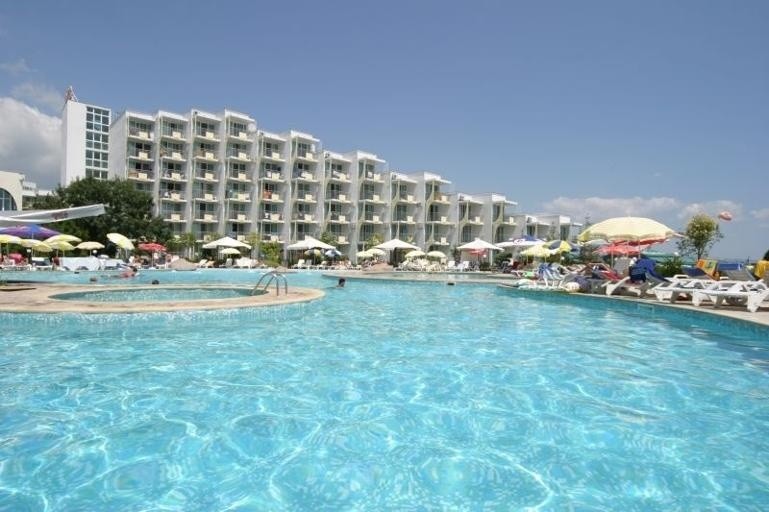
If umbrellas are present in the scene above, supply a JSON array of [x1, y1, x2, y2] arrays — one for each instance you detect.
[[496, 214, 686, 273], [0, 224, 168, 267]]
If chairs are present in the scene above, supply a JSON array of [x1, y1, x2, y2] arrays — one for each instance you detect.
[[0, 254, 362, 271], [361, 253, 769, 313]]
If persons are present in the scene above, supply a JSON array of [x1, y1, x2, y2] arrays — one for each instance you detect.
[[111, 268, 138, 278], [336, 278, 345, 287], [753, 250, 769, 280]]
